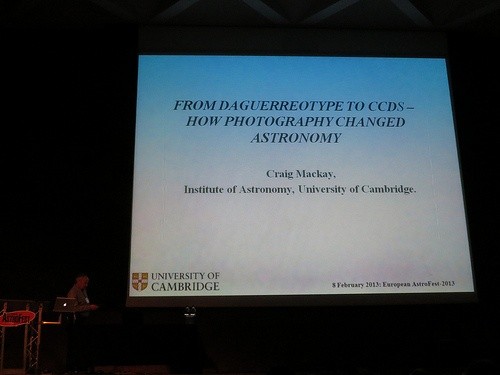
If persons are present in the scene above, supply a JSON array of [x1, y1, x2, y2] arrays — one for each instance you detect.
[[65, 273, 99, 375]]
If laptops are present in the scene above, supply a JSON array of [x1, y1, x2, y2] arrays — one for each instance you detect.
[[53, 297, 77, 312]]
